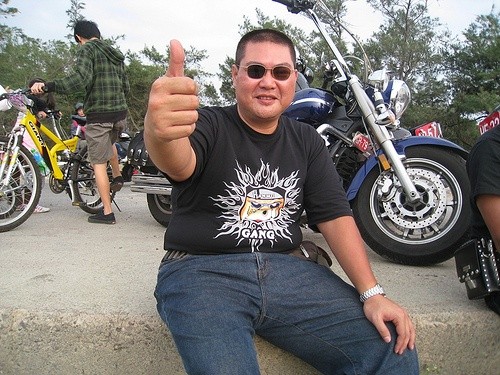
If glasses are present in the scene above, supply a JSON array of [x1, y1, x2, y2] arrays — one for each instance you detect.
[[235, 64, 297, 81]]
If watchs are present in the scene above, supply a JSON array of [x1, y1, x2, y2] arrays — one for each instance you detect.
[[360, 283, 385, 302]]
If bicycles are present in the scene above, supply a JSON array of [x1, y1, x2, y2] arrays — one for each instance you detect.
[[0, 86, 125, 234]]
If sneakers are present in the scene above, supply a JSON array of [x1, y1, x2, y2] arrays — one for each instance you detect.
[[87, 209, 117, 225], [111, 176, 124, 192]]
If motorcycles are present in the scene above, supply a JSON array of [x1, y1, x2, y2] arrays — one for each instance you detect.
[[127, 0, 479, 268]]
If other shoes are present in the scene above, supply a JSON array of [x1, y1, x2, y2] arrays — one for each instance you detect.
[[14, 204, 50, 214]]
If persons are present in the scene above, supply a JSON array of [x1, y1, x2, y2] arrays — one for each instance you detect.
[[71, 99, 86, 139], [142, 29, 419, 375], [465, 123, 500, 316], [29, 20, 132, 225], [7, 76, 63, 213]]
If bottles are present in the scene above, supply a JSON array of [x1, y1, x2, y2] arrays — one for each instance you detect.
[[31, 148, 51, 176]]
